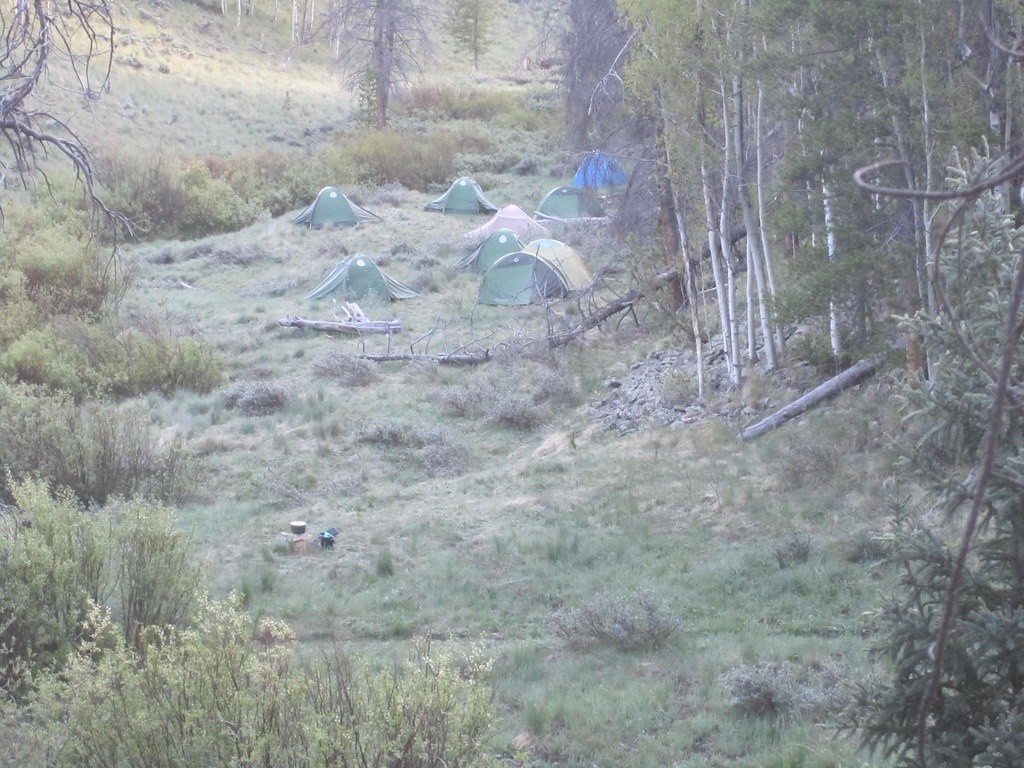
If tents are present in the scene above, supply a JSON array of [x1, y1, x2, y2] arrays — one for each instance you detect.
[[532, 185, 612, 222], [475, 238, 593, 307], [303, 252, 421, 305], [453, 227, 528, 276], [423, 175, 498, 214], [292, 186, 381, 226], [571, 148, 627, 187], [456, 203, 551, 242]]
